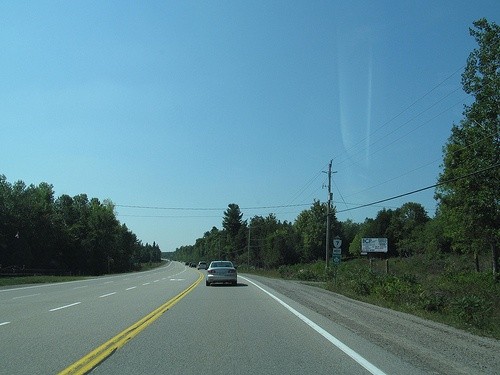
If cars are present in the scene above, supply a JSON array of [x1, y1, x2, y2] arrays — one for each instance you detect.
[[185, 261, 196, 268], [205, 261, 237, 286], [197, 261, 208, 270]]
